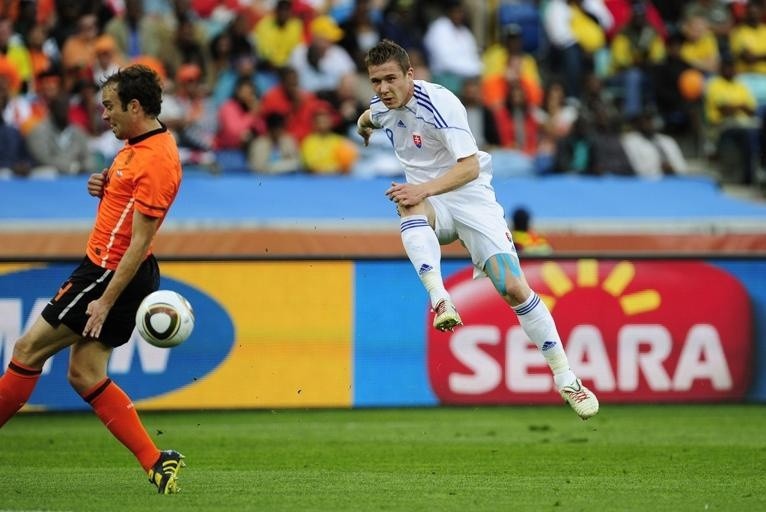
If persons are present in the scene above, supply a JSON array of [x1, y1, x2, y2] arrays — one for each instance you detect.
[[358, 40, 600, 422], [0, 0, 765, 196], [0, 64, 186, 494]]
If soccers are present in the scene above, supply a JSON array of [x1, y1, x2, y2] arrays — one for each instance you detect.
[[136, 290, 194, 347]]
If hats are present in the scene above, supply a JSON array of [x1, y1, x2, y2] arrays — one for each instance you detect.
[[308, 14, 345, 43]]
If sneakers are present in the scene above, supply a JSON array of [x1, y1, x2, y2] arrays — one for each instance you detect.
[[431, 298, 463, 333], [148, 450, 187, 495], [558, 376, 599, 421]]
